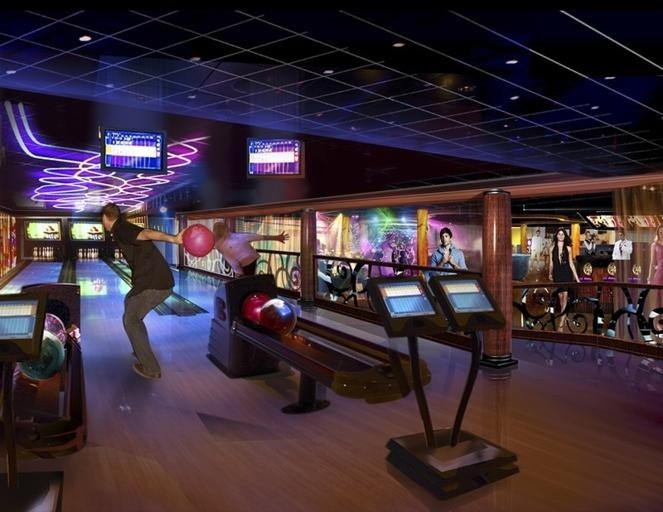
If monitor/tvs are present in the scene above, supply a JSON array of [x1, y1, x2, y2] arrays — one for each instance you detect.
[[439, 279, 496, 314], [377, 280, 437, 318], [245, 136, 305, 179], [0, 299, 39, 339], [101, 126, 167, 174]]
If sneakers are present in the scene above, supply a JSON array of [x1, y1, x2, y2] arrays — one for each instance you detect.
[[133, 364, 161, 379]]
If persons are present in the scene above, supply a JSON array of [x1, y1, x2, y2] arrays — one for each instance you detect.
[[646, 223, 662, 338], [315, 234, 417, 298], [587, 214, 662, 230], [99, 202, 193, 380], [581, 230, 597, 257], [611, 229, 633, 261], [211, 221, 291, 281], [428, 226, 469, 278], [547, 226, 584, 333], [529, 229, 546, 272]]
